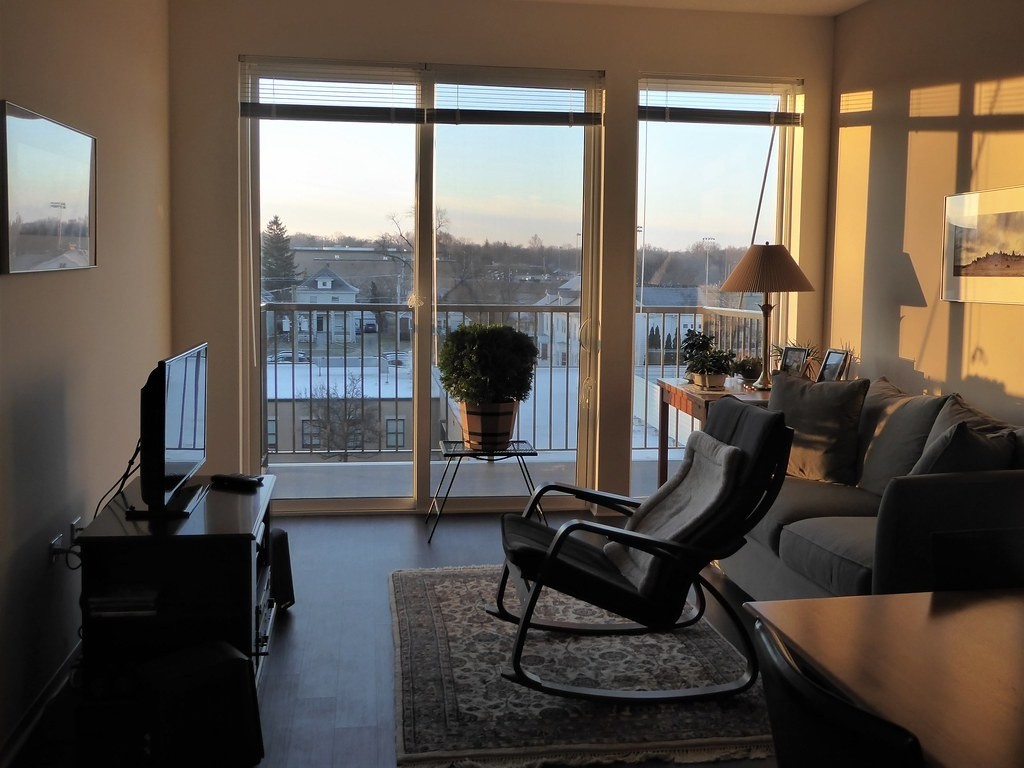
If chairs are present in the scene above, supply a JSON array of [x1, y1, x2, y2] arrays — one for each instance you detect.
[[753, 615, 925, 768], [930, 531, 1024, 590], [486, 394, 794, 703]]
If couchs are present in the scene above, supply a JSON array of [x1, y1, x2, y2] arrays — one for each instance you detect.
[[718, 375, 1024, 603]]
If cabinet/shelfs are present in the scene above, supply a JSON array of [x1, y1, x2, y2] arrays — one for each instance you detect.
[[77, 475, 278, 703]]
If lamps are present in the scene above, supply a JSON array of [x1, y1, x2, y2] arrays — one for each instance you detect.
[[719, 241, 816, 391]]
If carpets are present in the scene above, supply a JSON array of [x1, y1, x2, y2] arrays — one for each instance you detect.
[[388, 564, 776, 768]]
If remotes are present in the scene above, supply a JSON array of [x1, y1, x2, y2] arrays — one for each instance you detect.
[[211, 473, 264, 486]]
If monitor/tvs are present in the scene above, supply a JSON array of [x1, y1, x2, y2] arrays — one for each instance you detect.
[[126, 341, 210, 519]]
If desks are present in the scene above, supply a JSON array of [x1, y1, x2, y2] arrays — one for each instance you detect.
[[739, 589, 1024, 768], [426, 440, 548, 544], [656, 378, 771, 492]]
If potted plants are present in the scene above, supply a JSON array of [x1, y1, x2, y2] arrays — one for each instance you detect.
[[681, 328, 763, 391], [437, 323, 541, 451]]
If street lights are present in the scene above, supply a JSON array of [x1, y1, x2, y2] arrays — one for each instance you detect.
[[702, 237, 715, 305]]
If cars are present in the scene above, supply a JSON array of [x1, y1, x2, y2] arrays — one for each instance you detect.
[[365, 323, 376, 333], [267, 350, 309, 361]]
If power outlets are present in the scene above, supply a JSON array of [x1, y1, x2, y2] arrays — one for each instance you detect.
[[49, 533, 65, 565]]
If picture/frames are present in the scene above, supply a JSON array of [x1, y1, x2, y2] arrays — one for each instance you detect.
[[816, 349, 848, 383], [779, 347, 810, 374], [0, 99, 98, 273], [939, 184, 1024, 306]]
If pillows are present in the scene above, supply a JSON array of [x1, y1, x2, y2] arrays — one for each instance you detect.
[[767, 370, 869, 486], [904, 421, 1017, 475]]
